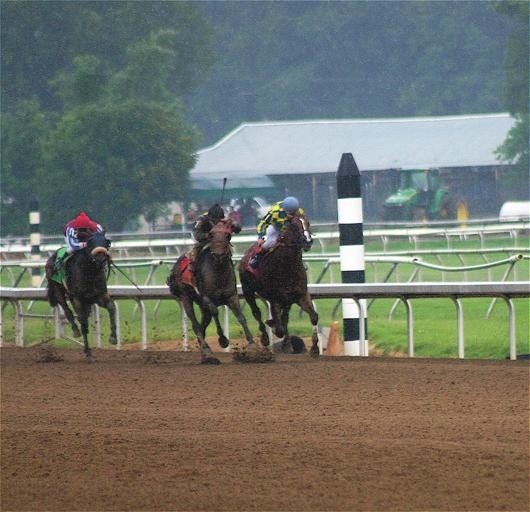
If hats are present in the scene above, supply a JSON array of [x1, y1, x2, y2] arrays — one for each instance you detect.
[[208, 204, 225, 219], [282, 196, 299, 211]]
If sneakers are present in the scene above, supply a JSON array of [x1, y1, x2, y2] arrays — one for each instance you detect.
[[249, 253, 262, 269]]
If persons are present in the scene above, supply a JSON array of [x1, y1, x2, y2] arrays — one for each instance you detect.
[[161, 205, 242, 229], [413, 195, 470, 232], [249, 197, 308, 271], [184, 204, 243, 272], [53, 212, 108, 271]]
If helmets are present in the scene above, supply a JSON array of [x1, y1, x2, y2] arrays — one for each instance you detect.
[[73, 212, 91, 229]]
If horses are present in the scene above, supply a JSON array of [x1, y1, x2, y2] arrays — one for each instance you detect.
[[166, 218, 255, 356], [239, 212, 319, 360], [45, 230, 117, 358]]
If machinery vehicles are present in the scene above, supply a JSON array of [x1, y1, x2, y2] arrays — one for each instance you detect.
[[382, 169, 470, 227]]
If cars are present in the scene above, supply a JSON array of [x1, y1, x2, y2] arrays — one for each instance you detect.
[[220, 196, 274, 226]]
